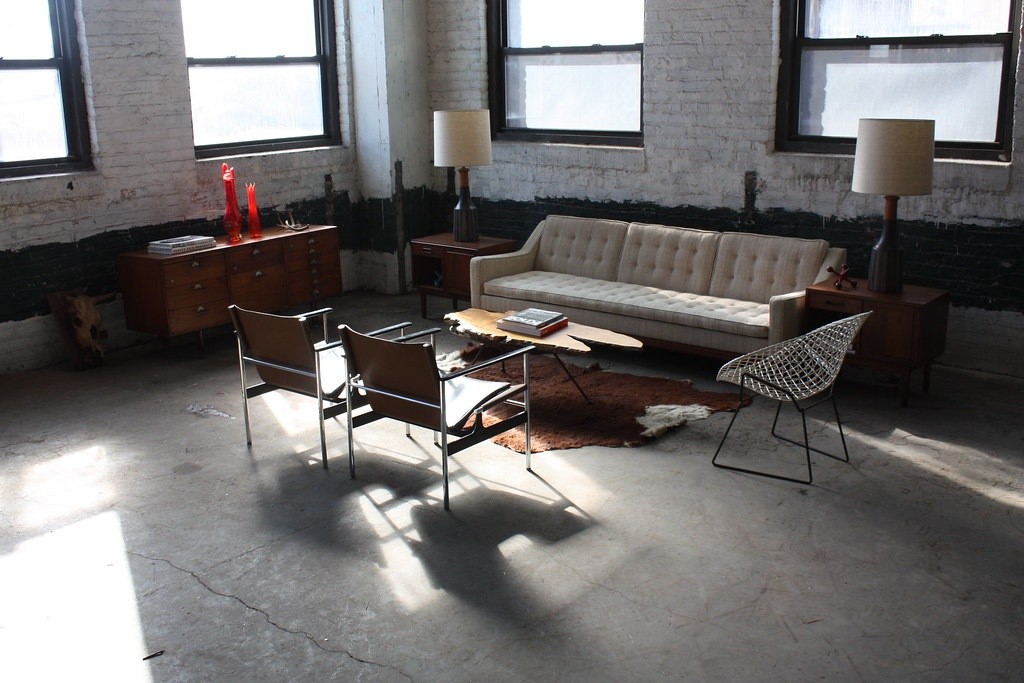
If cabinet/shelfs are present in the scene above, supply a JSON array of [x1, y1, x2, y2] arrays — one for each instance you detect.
[[214, 230, 287, 315], [266, 221, 344, 326], [116, 246, 232, 359]]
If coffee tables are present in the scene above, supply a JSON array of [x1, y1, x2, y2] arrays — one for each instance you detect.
[[442, 308, 644, 406]]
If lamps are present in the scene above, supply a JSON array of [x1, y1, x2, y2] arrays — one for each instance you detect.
[[851, 119, 935, 295], [432, 109, 494, 243]]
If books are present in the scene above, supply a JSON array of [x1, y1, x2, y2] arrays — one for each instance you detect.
[[147, 235, 217, 255], [496, 308, 569, 338]]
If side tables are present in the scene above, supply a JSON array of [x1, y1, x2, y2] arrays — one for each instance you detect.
[[805, 274, 951, 405], [410, 233, 516, 319]]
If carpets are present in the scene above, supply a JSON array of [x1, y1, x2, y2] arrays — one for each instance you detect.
[[437, 342, 753, 454]]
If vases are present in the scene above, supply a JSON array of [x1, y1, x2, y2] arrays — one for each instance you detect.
[[245, 183, 262, 238], [221, 162, 244, 242]]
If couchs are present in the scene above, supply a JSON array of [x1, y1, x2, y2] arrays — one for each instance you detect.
[[470, 214, 847, 367]]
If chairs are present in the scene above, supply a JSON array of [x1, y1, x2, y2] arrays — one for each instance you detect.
[[712, 309, 874, 484], [228, 304, 413, 470], [337, 324, 538, 510]]
